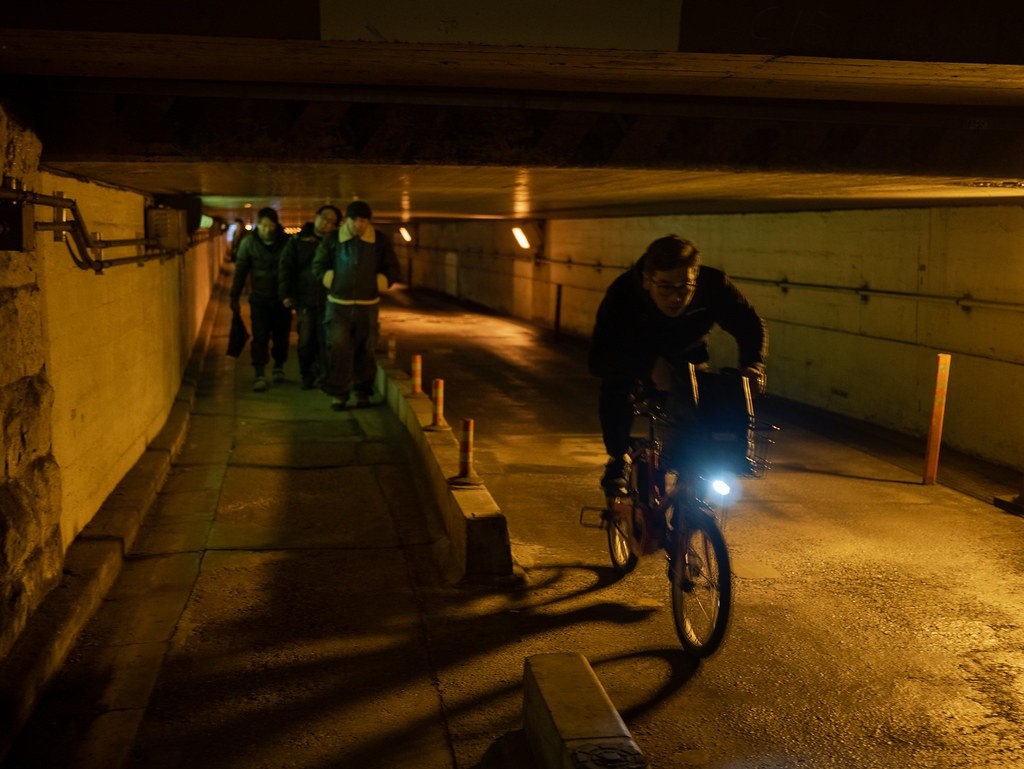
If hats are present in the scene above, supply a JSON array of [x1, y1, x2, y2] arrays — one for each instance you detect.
[[345, 200, 371, 214]]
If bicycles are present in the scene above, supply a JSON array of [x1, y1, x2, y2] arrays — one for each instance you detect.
[[580, 369, 779, 657]]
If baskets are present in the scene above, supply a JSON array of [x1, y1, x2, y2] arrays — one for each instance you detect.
[[661, 408, 780, 481]]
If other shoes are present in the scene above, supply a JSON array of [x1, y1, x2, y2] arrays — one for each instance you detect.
[[272, 368, 284, 381], [664, 540, 692, 592], [253, 376, 268, 389], [357, 396, 370, 408], [599, 453, 632, 488], [301, 377, 314, 389], [332, 396, 346, 407]]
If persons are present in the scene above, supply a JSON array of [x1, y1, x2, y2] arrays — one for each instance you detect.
[[229, 207, 292, 390], [231, 217, 250, 263], [312, 200, 402, 413], [590, 234, 769, 580], [278, 204, 342, 392]]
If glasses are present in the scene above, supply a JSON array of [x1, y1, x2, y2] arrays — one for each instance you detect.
[[650, 278, 696, 296], [318, 213, 337, 224]]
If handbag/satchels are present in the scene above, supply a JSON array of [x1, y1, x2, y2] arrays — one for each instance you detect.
[[675, 360, 756, 474], [225, 306, 251, 358]]
